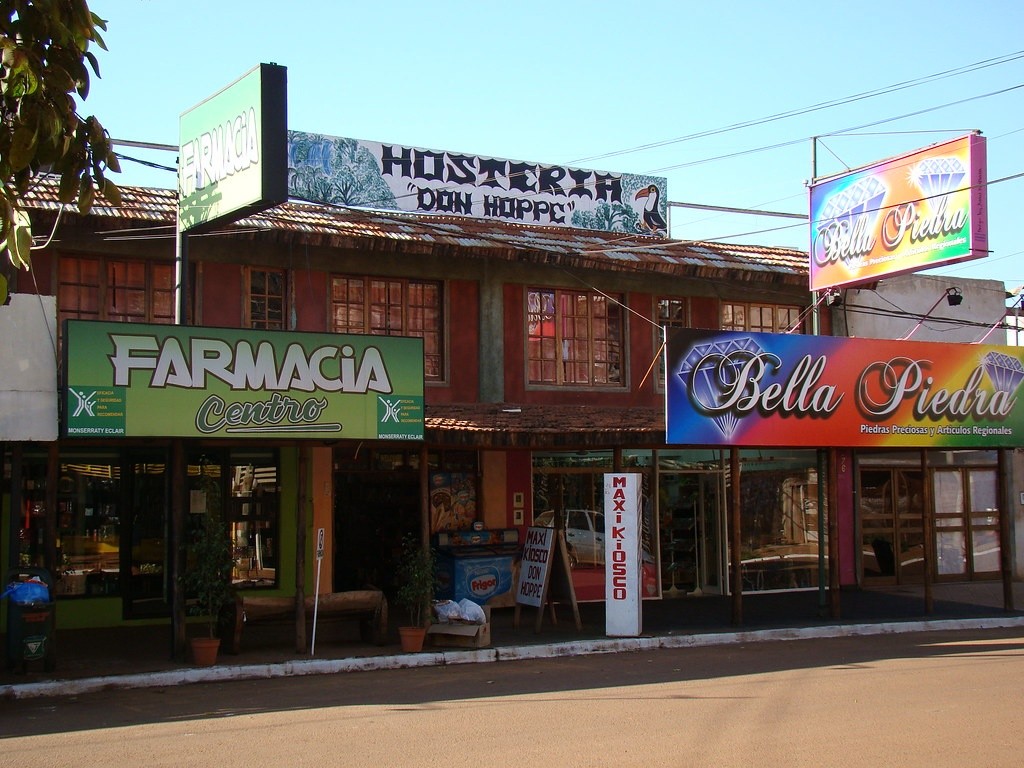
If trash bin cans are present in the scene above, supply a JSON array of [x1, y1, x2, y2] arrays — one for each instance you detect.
[[7, 567, 56, 675]]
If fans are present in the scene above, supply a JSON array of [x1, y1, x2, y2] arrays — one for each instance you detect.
[[548, 451, 614, 455]]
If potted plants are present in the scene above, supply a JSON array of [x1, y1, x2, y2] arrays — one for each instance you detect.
[[176, 473, 235, 668], [394, 530, 441, 653]]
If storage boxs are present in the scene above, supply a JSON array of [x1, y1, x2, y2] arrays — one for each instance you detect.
[[428, 605, 491, 648]]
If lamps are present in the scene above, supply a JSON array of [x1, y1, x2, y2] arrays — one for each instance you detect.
[[903, 286, 964, 341], [787, 289, 842, 332], [969, 294, 1024, 345]]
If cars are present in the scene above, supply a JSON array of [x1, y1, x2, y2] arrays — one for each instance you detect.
[[534, 509, 651, 571]]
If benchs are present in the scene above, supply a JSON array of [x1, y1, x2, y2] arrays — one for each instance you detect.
[[233, 590, 390, 653]]
